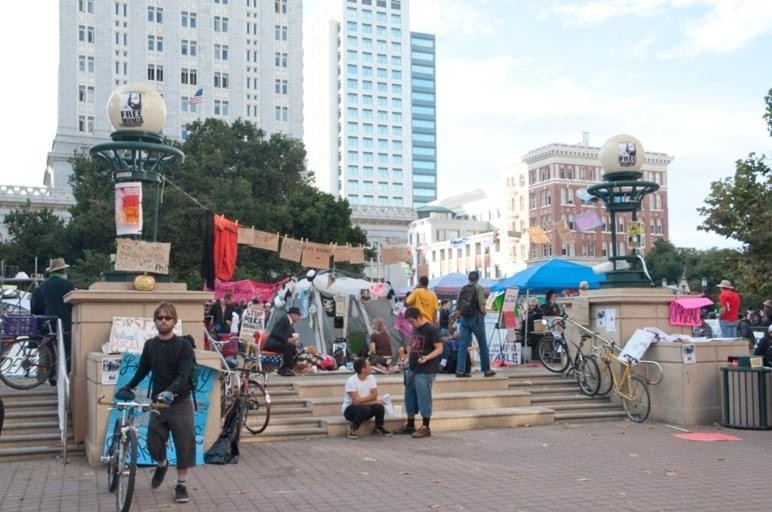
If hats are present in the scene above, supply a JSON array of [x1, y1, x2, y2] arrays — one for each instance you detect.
[[287, 307, 300, 314], [46, 258, 70, 273], [768, 325, 772, 333], [716, 279, 734, 289], [762, 300, 772, 308]]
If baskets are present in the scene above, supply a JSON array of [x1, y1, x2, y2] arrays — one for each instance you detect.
[[7, 315, 36, 336]]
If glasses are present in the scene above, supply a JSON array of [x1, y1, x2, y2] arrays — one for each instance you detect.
[[154, 315, 174, 321]]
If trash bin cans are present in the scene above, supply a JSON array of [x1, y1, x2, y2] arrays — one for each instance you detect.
[[720, 355, 772, 430]]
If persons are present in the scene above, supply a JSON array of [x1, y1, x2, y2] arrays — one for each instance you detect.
[[697, 297, 772, 368], [29, 256, 76, 387], [391, 306, 444, 437], [625, 143, 636, 156], [123, 92, 142, 112], [115, 300, 197, 503], [209, 270, 568, 377], [628, 226, 641, 243], [342, 358, 393, 439], [715, 279, 741, 338]]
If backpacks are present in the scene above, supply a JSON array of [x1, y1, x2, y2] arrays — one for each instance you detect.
[[459, 285, 477, 316]]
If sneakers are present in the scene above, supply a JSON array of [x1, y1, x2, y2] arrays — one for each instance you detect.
[[484, 369, 497, 376], [346, 421, 358, 438], [175, 484, 190, 502], [374, 426, 430, 437], [151, 458, 168, 488], [277, 367, 294, 376], [456, 371, 471, 377]]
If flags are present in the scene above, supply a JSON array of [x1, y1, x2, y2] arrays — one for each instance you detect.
[[575, 210, 603, 232], [527, 225, 549, 245], [553, 220, 575, 243], [186, 87, 204, 106]]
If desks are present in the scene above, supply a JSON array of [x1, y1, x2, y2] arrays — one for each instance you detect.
[[515, 329, 565, 360]]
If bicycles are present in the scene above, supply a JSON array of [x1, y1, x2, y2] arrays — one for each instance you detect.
[[529, 312, 602, 404], [214, 338, 277, 439], [90, 391, 167, 509], [591, 338, 654, 425], [3, 326, 72, 393]]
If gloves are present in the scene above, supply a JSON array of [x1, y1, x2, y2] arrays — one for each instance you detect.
[[158, 391, 173, 403]]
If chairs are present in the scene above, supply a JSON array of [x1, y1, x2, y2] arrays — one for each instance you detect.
[[251, 331, 282, 379]]
